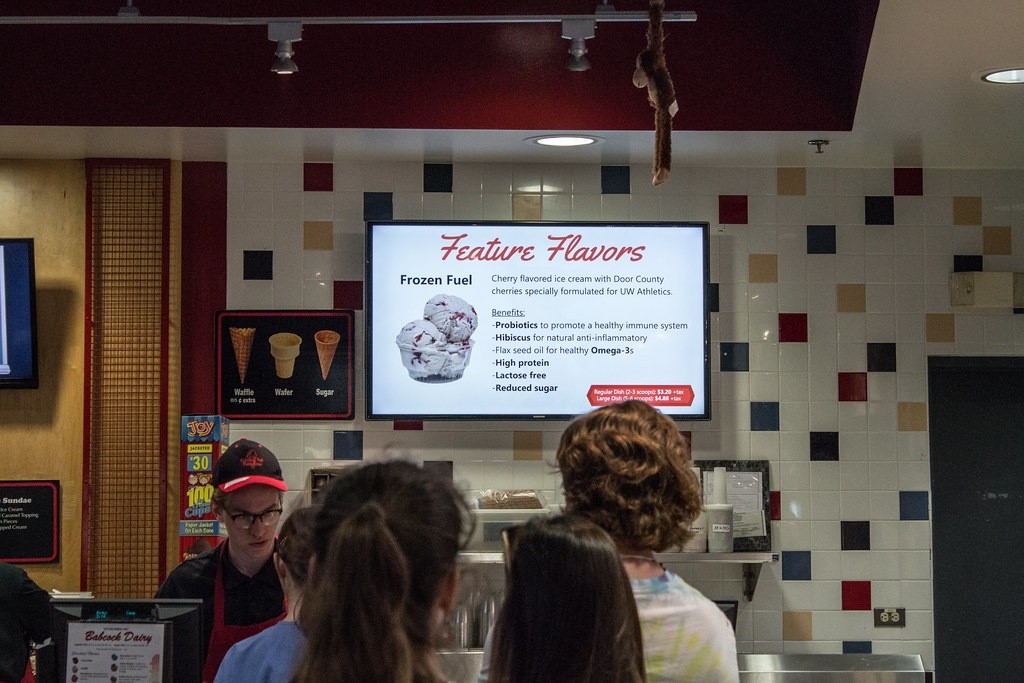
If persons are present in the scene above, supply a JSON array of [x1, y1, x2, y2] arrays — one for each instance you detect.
[[0, 561, 60, 683], [154, 438, 297, 682], [211, 457, 478, 683], [486, 511, 647, 683], [557, 400, 742, 683]]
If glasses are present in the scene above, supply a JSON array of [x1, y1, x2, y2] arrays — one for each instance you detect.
[[222, 497, 283, 530]]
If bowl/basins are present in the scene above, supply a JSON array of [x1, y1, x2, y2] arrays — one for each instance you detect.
[[397, 335, 475, 383]]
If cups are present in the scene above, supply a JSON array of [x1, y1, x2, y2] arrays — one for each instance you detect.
[[706, 505, 735, 553], [435, 652, 484, 683], [683, 506, 706, 553]]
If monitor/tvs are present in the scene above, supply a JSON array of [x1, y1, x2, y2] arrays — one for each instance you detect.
[[361, 217, 709, 421], [44, 597, 205, 683]]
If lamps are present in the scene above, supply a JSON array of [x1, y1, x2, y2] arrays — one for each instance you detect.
[[561, 20, 595, 71], [267, 22, 302, 73]]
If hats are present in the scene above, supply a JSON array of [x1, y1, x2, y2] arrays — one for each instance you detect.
[[212, 437, 287, 492]]
